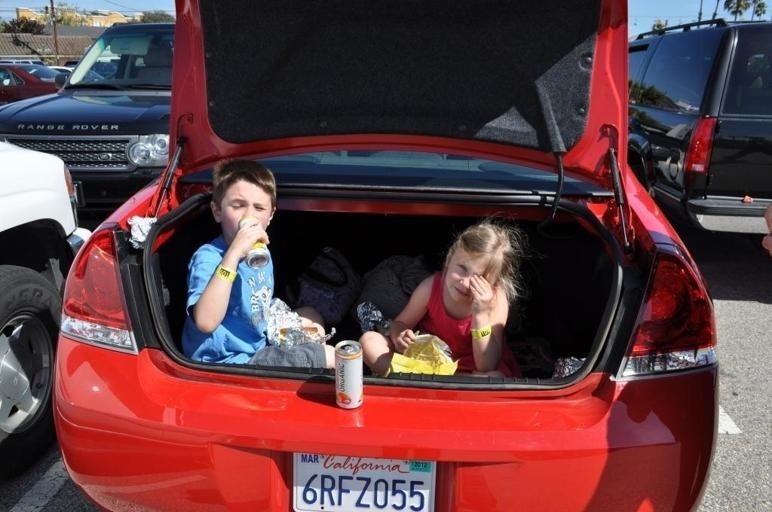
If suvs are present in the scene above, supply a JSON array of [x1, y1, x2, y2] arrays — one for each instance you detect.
[[628, 18, 772, 265], [0, 140, 94, 483], [0, 21, 175, 221]]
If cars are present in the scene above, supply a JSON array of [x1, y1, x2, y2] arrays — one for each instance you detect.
[[50, 0, 722, 512], [0, 59, 119, 105]]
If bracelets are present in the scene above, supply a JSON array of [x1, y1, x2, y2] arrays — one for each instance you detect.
[[468, 323, 493, 340], [214, 264, 240, 283]]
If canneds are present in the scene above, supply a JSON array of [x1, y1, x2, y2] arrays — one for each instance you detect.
[[334, 339, 363, 409], [238, 216, 270, 269]]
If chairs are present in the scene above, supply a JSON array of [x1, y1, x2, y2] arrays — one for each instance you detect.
[[136, 39, 173, 84]]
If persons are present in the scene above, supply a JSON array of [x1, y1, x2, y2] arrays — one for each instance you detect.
[[359, 222, 524, 379], [181, 158, 338, 370], [757, 199, 772, 260]]
